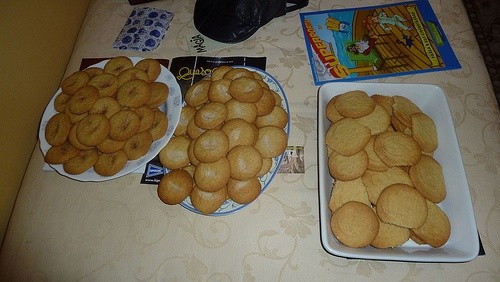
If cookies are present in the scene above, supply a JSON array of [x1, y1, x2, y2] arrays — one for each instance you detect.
[[325, 89, 450, 249], [157, 66, 288, 216], [45, 56, 168, 176]]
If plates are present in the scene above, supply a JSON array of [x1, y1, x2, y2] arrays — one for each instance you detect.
[[38, 57, 182, 183], [163, 64, 290, 217]]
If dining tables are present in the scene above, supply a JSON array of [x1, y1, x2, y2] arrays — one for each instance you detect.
[[1, 0, 499, 281]]
